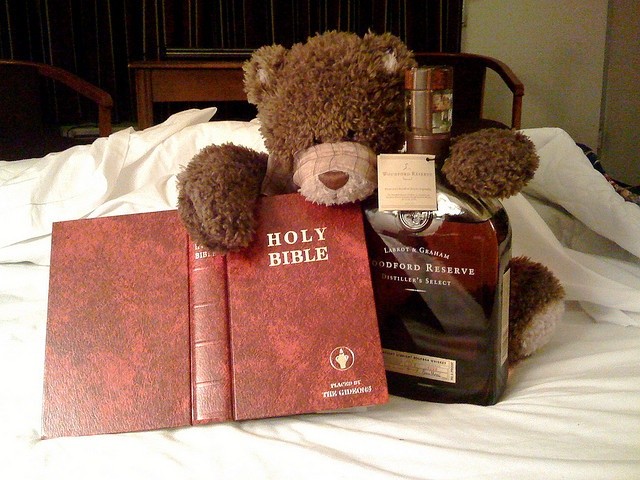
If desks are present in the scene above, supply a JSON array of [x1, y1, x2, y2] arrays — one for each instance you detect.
[[128, 50, 255, 129]]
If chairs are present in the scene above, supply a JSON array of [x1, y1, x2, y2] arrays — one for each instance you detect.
[[408, 52, 523, 138], [0, 61, 114, 163]]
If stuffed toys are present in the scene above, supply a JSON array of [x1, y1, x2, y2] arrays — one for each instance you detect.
[[176, 28, 567, 373]]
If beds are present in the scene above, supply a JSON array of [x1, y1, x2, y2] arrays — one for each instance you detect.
[[0, 107, 640, 480]]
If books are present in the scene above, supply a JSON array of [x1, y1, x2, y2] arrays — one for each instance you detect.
[[42, 192, 389, 440]]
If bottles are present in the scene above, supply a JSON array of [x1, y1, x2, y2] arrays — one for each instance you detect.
[[361, 65, 512, 406]]
